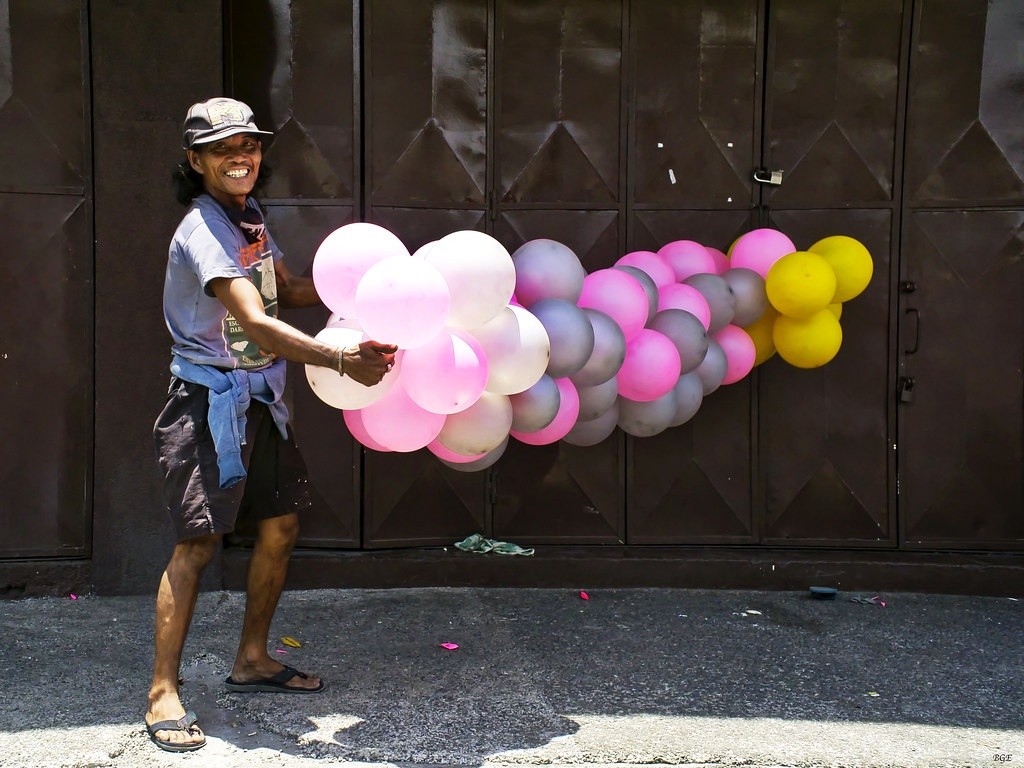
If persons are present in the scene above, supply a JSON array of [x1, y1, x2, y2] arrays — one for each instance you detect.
[[141, 96, 330, 752]]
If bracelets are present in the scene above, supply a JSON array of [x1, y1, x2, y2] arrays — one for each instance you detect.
[[337, 346, 347, 376]]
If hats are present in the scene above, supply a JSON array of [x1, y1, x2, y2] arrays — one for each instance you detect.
[[180, 97, 274, 149]]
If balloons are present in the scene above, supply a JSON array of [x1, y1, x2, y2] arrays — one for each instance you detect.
[[304, 221, 874, 471]]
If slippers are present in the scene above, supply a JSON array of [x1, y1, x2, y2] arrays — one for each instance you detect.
[[145, 708, 207, 752], [225, 665, 326, 694]]
